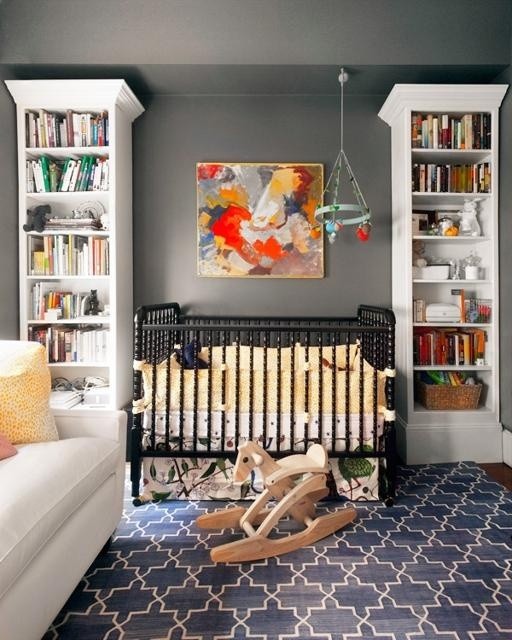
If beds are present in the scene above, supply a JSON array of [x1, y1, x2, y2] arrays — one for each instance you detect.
[[131, 299, 400, 511]]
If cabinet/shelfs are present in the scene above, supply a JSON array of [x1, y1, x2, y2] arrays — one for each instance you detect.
[[1, 77, 147, 411], [376, 79, 510, 466]]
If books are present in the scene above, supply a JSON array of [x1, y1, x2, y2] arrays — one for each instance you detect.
[[412, 111, 491, 365], [25, 107, 108, 364]]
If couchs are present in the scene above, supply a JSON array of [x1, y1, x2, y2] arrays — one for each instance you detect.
[[1, 411, 129, 640]]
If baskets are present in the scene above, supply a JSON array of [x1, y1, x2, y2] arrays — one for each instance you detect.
[[416, 379, 483, 410]]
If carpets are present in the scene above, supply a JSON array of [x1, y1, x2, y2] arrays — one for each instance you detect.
[[44, 460, 512, 640]]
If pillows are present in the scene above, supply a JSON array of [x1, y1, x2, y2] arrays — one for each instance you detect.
[[0, 338, 62, 446]]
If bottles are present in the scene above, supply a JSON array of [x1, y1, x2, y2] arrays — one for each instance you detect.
[[448, 254, 482, 279], [428, 208, 482, 236]]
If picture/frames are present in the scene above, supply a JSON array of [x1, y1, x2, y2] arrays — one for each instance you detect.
[[195, 161, 326, 280], [434, 208, 460, 226]]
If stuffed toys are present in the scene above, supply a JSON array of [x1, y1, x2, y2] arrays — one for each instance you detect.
[[303, 218, 372, 243], [173, 338, 207, 368]]
